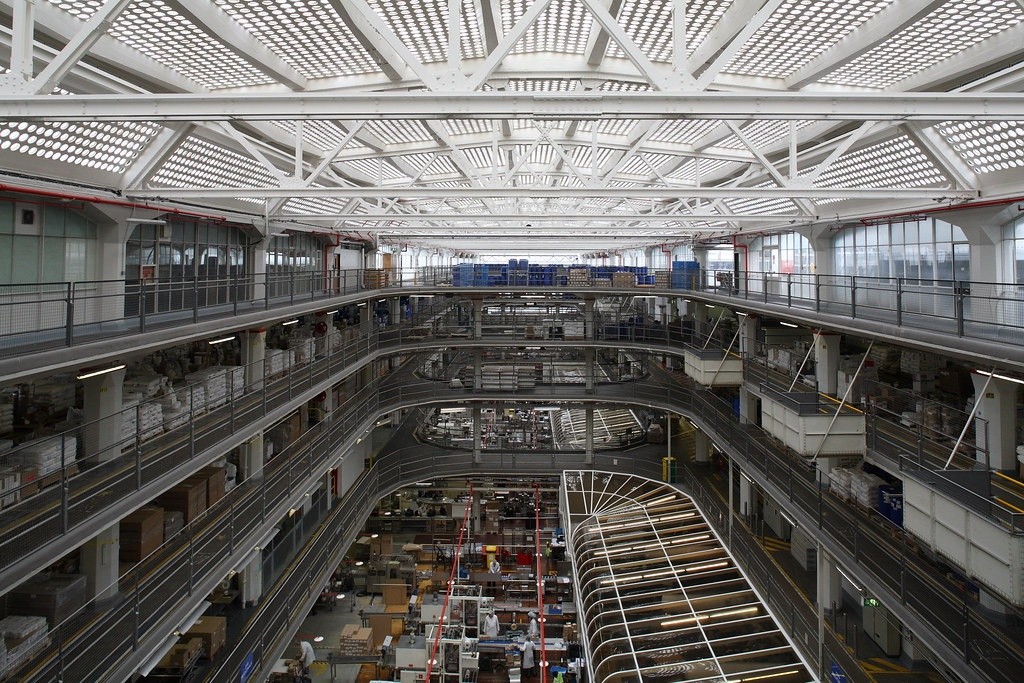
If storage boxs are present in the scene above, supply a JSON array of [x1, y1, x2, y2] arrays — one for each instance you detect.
[[452, 259, 700, 290], [177, 644, 196, 659], [285, 660, 299, 673], [169, 649, 189, 673], [178, 616, 226, 658], [339, 623, 374, 656]]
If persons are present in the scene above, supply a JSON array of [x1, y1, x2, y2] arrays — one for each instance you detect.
[[518, 637, 536, 677], [483, 610, 500, 636], [294, 639, 315, 668], [527, 611, 539, 637], [532, 490, 541, 500], [418, 504, 426, 517], [488, 560, 500, 573]]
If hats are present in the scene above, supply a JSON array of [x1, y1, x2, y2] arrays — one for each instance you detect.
[[488, 610, 494, 616], [493, 559, 496, 563], [528, 612, 536, 618], [525, 634, 531, 639]]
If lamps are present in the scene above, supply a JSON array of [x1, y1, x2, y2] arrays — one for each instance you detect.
[[271, 222, 289, 238], [125, 205, 166, 225]]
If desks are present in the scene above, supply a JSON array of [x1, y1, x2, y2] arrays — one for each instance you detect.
[[361, 613, 404, 632], [212, 590, 242, 626], [330, 656, 380, 683]]
